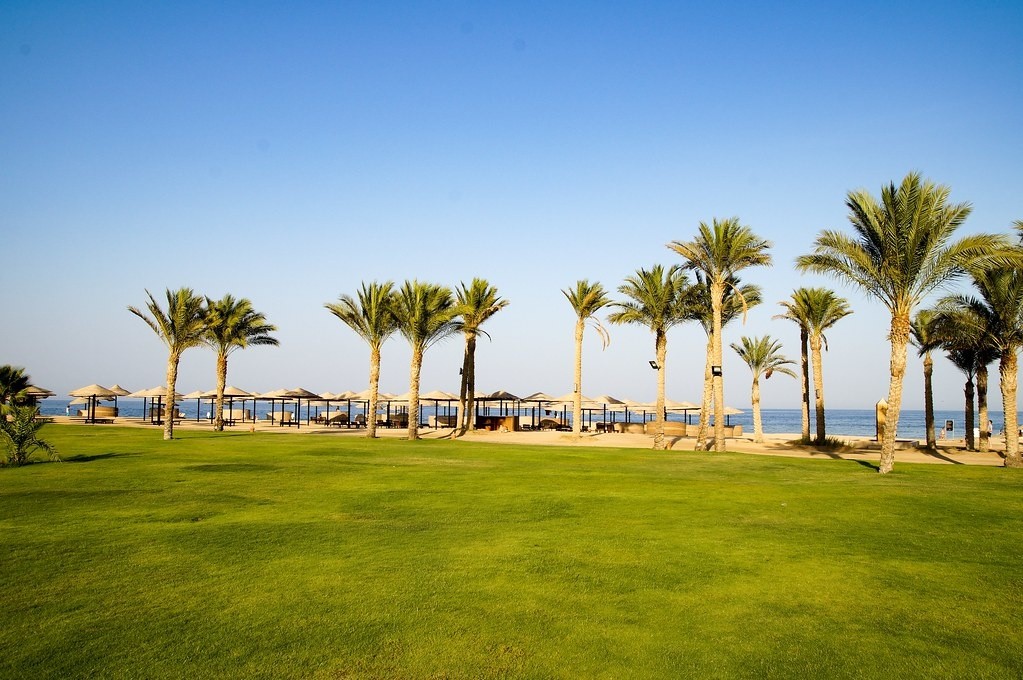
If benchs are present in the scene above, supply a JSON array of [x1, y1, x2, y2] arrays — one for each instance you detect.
[[29, 406, 743, 440], [869, 440, 912, 450]]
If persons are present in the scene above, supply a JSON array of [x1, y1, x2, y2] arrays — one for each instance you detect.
[[66, 405, 70, 416], [987, 419, 993, 449], [939, 426, 946, 440], [319, 414, 322, 423], [206, 412, 210, 419], [291, 412, 295, 420]]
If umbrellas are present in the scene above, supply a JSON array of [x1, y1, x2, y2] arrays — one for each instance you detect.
[[145, 385, 186, 422], [17, 385, 56, 409], [128, 388, 152, 421], [200, 377, 752, 452], [183, 390, 210, 423], [68, 383, 131, 422]]
